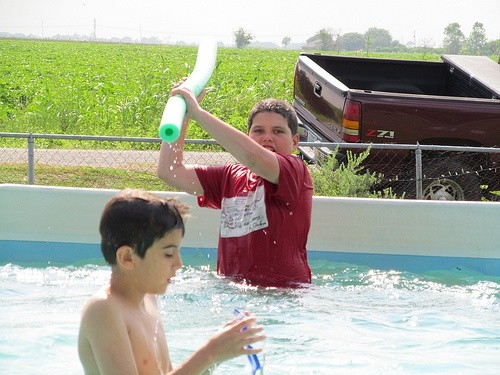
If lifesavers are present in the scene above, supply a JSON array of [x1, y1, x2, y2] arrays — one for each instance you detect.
[[160, 39, 216, 143]]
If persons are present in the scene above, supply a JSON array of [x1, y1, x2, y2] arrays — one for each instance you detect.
[[156, 76, 313, 288], [78, 189, 265, 375]]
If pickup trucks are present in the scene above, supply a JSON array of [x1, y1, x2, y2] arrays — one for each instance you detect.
[[291, 53, 500, 201]]
